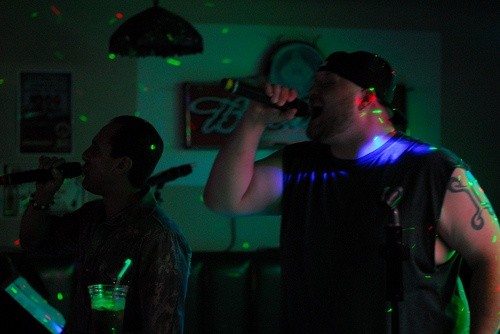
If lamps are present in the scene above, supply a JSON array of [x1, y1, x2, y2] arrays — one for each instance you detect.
[[108, 0, 204, 58]]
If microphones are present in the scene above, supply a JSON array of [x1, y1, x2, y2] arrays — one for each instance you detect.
[[0, 161, 83, 186], [221, 77, 312, 120]]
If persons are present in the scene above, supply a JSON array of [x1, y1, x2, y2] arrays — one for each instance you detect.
[[19, 115, 190, 334], [204, 51, 500, 334]]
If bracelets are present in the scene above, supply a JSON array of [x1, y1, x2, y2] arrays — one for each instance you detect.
[[29, 193, 56, 209]]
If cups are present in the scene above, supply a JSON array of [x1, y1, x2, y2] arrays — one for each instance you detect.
[[88, 284, 129, 334]]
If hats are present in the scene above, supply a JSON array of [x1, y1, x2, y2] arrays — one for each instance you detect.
[[318, 51, 395, 106]]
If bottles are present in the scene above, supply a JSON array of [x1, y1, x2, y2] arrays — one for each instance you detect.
[[3, 185, 18, 217]]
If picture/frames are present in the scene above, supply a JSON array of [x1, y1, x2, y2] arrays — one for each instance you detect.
[[15, 69, 75, 158]]
